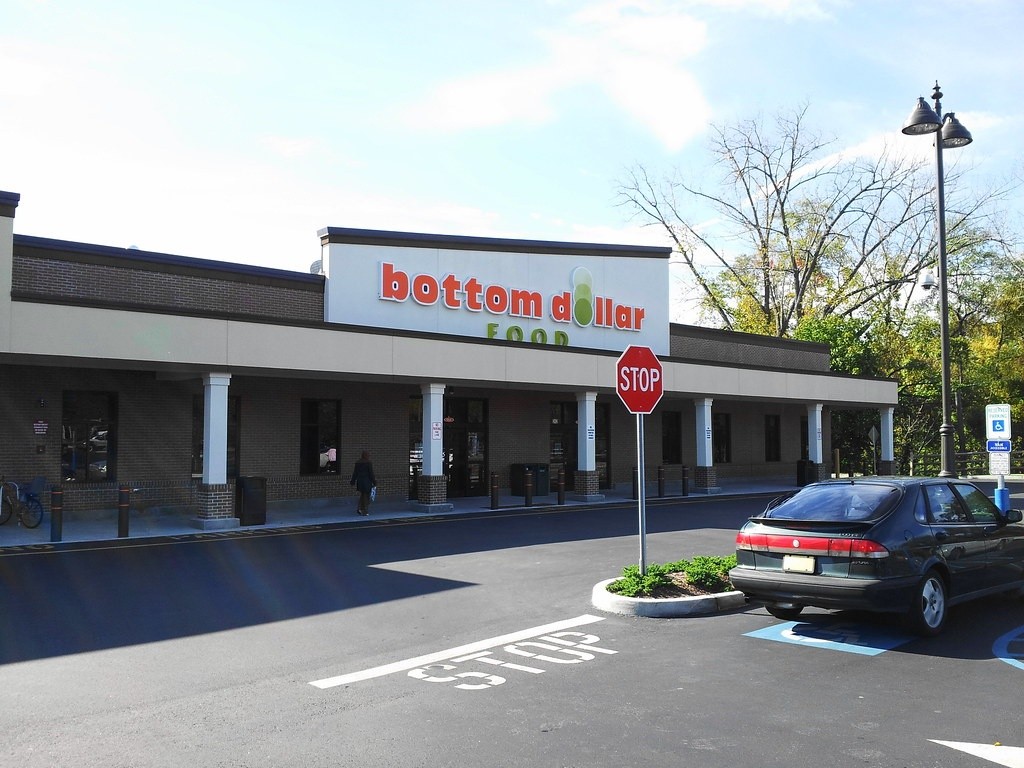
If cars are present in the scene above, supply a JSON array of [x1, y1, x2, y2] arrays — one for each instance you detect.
[[728, 475, 1024, 638], [63, 427, 109, 482]]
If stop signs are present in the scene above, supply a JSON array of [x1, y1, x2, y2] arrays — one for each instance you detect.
[[616, 343, 664, 415]]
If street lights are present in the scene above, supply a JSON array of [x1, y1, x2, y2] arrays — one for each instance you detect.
[[899, 77, 974, 509]]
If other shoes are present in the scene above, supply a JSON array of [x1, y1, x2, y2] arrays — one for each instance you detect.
[[363, 513, 369, 516], [357, 509, 363, 516]]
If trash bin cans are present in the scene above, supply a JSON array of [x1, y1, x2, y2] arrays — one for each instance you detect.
[[796, 459, 814, 487], [234, 475, 268, 526], [510, 462, 549, 496]]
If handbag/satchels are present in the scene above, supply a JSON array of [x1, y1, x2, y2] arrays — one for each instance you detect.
[[368, 486, 377, 504]]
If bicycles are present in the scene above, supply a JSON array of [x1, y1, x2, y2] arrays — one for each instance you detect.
[[0, 475, 44, 529]]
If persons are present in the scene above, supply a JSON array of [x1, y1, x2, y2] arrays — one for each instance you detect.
[[349, 450, 377, 516]]
